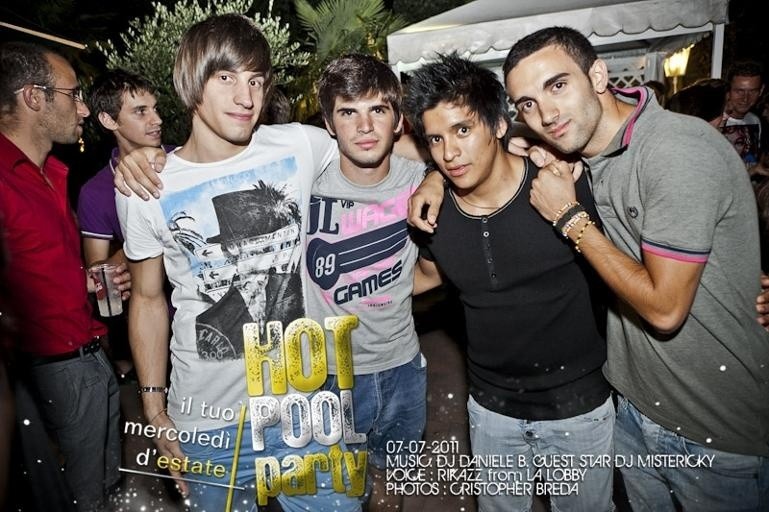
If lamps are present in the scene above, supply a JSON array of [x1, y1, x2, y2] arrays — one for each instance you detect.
[[664, 45, 691, 78]]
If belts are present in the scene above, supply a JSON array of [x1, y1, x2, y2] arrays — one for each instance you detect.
[[32, 337, 102, 367]]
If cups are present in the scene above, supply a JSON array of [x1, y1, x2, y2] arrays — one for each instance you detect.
[[88, 264, 124, 318]]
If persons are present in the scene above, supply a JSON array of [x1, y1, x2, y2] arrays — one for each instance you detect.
[[1, 10, 768, 510]]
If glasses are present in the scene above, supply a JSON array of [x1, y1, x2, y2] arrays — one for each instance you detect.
[[13, 85, 84, 104]]
